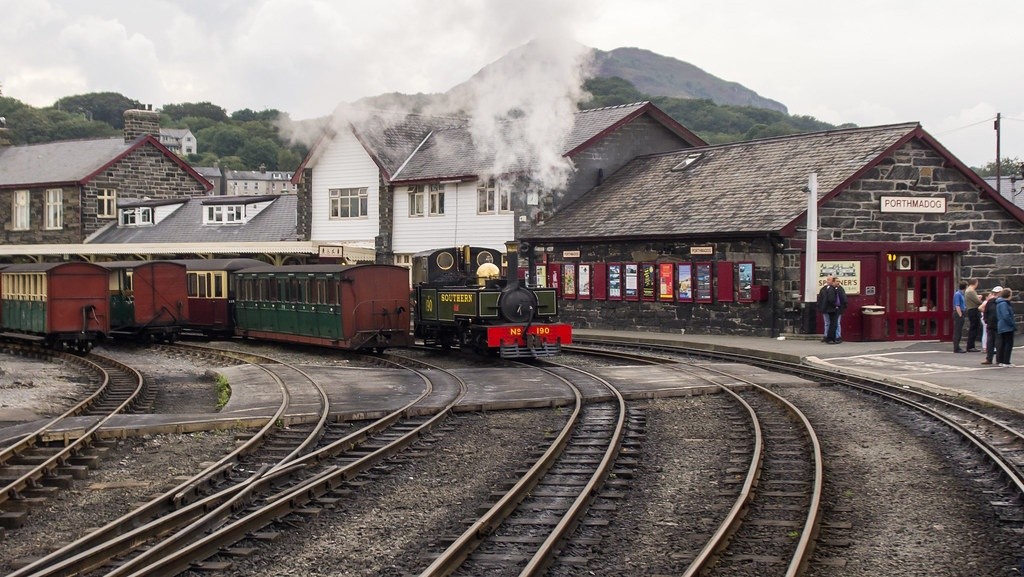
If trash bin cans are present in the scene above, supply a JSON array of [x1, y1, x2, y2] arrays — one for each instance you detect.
[[860, 303, 886, 341]]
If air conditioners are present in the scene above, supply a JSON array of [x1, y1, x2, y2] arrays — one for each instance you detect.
[[896, 255, 911, 270]]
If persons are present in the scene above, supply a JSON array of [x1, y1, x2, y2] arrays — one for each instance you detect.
[[952, 277, 1018, 370], [816, 276, 848, 344]]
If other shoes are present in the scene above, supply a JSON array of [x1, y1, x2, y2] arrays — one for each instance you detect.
[[982, 361, 992, 364], [999, 363, 1003, 366], [968, 348, 981, 352], [955, 349, 966, 353], [828, 340, 840, 344], [1003, 364, 1016, 367]]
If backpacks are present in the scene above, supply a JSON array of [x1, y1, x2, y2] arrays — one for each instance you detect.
[[818, 288, 832, 311]]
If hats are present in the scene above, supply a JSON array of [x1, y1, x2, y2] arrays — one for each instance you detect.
[[992, 286, 1003, 293]]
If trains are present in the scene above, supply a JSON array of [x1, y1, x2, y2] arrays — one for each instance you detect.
[[0, 241, 572, 359]]
